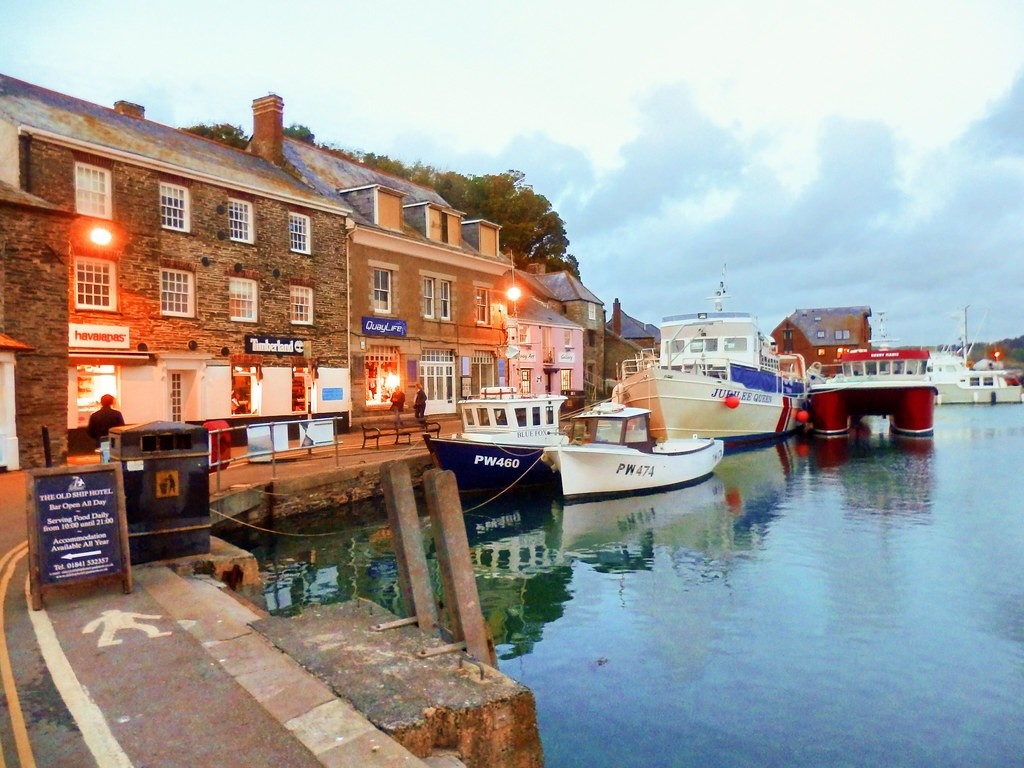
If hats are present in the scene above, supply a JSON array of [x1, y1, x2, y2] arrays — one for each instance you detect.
[[102, 395, 113, 405]]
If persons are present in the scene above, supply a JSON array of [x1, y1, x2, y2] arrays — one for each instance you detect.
[[87, 394, 124, 464], [413, 384, 429, 429], [389, 385, 408, 430]]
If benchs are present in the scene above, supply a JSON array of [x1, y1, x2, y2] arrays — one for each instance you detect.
[[361, 416, 441, 451]]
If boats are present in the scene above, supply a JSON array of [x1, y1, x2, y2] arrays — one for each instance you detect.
[[421, 386, 726, 501], [612, 261, 809, 454], [807, 310, 938, 442], [930, 303, 1024, 405]]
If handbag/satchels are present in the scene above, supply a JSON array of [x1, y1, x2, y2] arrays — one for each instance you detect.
[[413, 404, 417, 409], [392, 398, 397, 402]]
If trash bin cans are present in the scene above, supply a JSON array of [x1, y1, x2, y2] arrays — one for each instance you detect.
[[107, 420, 209, 564]]
